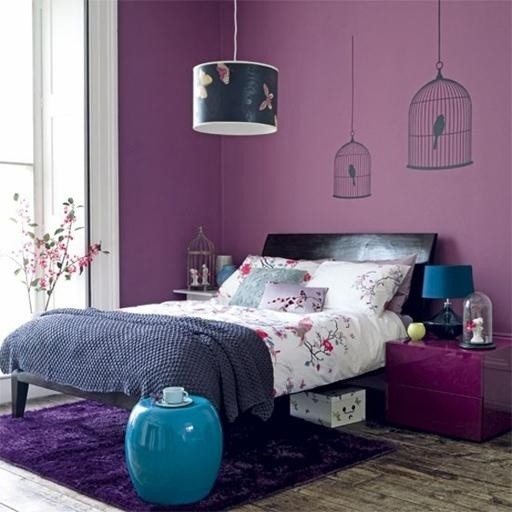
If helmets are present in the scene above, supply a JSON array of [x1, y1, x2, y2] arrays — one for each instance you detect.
[[156, 397, 193, 408]]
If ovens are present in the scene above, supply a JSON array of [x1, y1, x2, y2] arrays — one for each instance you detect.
[[0, 232, 438, 465]]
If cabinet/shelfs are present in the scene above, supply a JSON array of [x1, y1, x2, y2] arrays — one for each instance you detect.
[[215, 253, 237, 289]]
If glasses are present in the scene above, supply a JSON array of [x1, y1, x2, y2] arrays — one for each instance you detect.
[[417, 262, 477, 339], [189, 1, 281, 138]]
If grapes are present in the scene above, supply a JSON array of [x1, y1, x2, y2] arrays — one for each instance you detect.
[[173, 289, 220, 304], [383, 333, 511, 444]]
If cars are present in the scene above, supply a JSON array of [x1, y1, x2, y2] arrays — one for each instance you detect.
[[408, 322, 426, 340], [164, 386, 188, 404]]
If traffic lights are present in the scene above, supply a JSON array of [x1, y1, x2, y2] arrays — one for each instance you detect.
[[216, 254, 417, 322]]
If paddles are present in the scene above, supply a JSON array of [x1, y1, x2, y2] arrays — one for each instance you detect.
[[0, 396, 400, 511]]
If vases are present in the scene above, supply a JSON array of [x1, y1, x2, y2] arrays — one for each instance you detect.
[[8, 194, 113, 313]]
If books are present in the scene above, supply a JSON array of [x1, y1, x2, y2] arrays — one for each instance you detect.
[[288, 388, 367, 430]]
